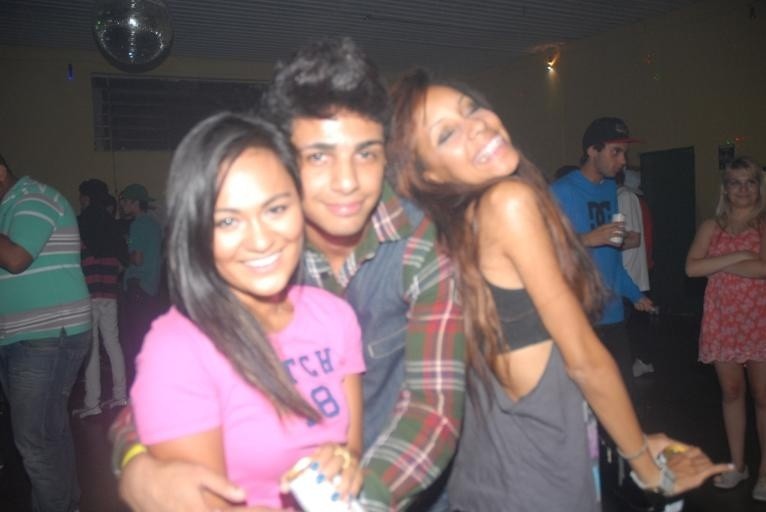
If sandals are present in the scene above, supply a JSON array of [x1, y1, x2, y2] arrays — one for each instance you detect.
[[752, 473, 766, 501], [713, 464, 751, 489]]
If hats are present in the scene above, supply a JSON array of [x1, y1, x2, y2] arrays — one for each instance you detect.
[[582, 116, 644, 152], [121, 183, 159, 203]]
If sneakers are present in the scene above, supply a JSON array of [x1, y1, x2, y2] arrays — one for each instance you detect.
[[71, 403, 103, 419], [103, 397, 129, 410]]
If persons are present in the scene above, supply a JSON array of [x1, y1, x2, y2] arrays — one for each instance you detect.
[[109, 37, 467, 512], [118, 182, 163, 398], [71, 177, 128, 419], [613, 169, 654, 376], [0, 154, 94, 512], [684, 157, 766, 502], [383, 66, 735, 512], [551, 117, 655, 404], [128, 110, 367, 512]]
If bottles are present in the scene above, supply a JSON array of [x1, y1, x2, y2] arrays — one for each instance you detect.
[[610, 214, 626, 243], [286, 456, 365, 512]]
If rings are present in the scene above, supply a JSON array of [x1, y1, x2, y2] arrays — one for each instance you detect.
[[332, 447, 352, 470]]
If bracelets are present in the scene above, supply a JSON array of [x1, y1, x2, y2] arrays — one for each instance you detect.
[[628, 460, 677, 495], [120, 442, 148, 470], [616, 434, 651, 461]]
